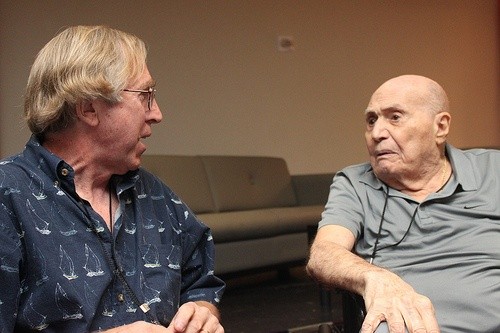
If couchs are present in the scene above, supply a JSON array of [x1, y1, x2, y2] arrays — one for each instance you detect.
[[139, 155, 336, 281]]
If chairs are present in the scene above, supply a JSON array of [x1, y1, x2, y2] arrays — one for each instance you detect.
[[342, 288, 366, 333]]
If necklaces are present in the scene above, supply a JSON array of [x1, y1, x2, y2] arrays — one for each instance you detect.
[[370, 170, 452, 265], [62, 180, 160, 325]]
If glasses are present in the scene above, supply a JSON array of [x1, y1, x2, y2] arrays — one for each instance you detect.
[[121, 84, 156, 110]]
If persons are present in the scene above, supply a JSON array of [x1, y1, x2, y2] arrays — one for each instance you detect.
[[306, 73, 500, 333], [0, 25, 225, 333]]
